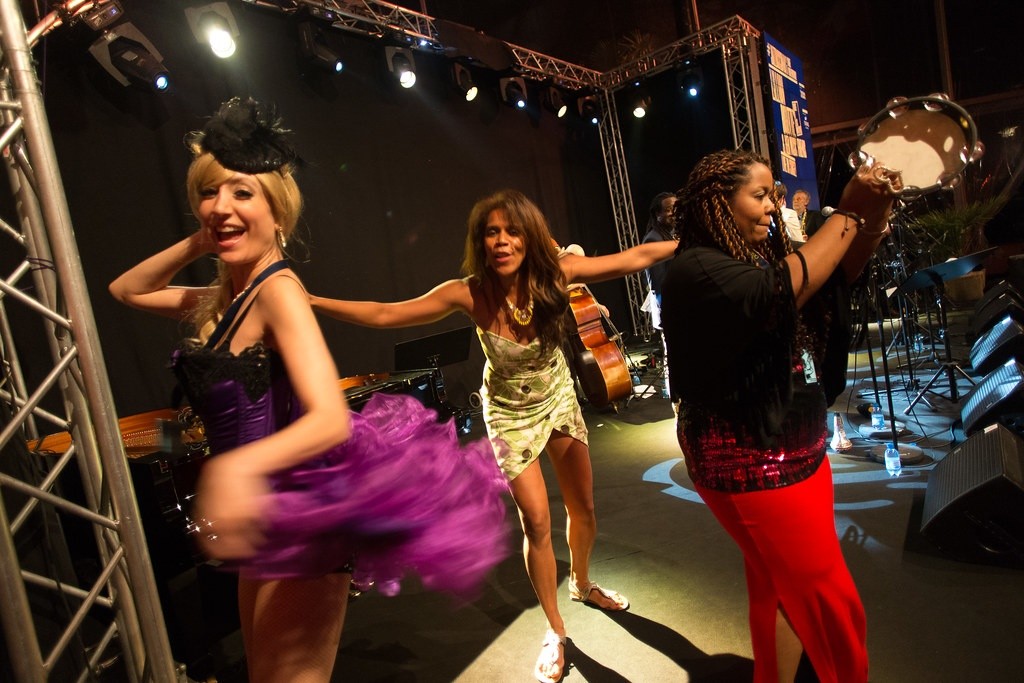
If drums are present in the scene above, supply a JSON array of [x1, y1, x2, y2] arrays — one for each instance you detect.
[[854, 97, 978, 195]]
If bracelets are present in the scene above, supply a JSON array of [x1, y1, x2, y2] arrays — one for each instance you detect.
[[861, 221, 891, 236], [821, 206, 867, 239]]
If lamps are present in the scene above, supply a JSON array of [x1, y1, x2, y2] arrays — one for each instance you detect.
[[544, 86, 567, 118], [381, 45, 418, 89], [498, 75, 528, 112], [88, 22, 172, 96], [185, 1, 241, 57], [630, 91, 650, 118], [578, 94, 599, 124], [677, 67, 706, 100], [301, 21, 343, 73]]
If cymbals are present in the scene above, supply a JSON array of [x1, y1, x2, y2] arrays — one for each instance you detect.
[[848, 91, 985, 202]]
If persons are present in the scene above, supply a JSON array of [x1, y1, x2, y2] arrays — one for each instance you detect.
[[106, 96, 514, 683], [775, 179, 806, 243], [643, 192, 681, 418], [306, 191, 681, 683], [669, 148, 901, 683], [793, 188, 826, 238]]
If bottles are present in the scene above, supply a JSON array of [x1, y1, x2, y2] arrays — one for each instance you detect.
[[915, 335, 923, 351], [661, 380, 668, 399], [884, 443, 901, 476], [633, 368, 640, 385], [830, 412, 852, 453], [871, 403, 884, 430]]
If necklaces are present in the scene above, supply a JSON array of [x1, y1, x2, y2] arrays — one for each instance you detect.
[[501, 278, 534, 326], [225, 262, 274, 312]]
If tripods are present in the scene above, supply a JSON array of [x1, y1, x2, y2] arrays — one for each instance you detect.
[[856, 204, 977, 415]]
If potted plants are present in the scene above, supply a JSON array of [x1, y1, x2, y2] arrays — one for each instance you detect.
[[901, 195, 1006, 304]]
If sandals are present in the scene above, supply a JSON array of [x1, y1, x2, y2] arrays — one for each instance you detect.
[[534, 627, 566, 683], [569, 578, 629, 612]]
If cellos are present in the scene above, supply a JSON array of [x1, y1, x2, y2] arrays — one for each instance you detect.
[[549, 236, 632, 415]]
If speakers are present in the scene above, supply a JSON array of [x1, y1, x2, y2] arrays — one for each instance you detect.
[[920, 281, 1024, 559]]
